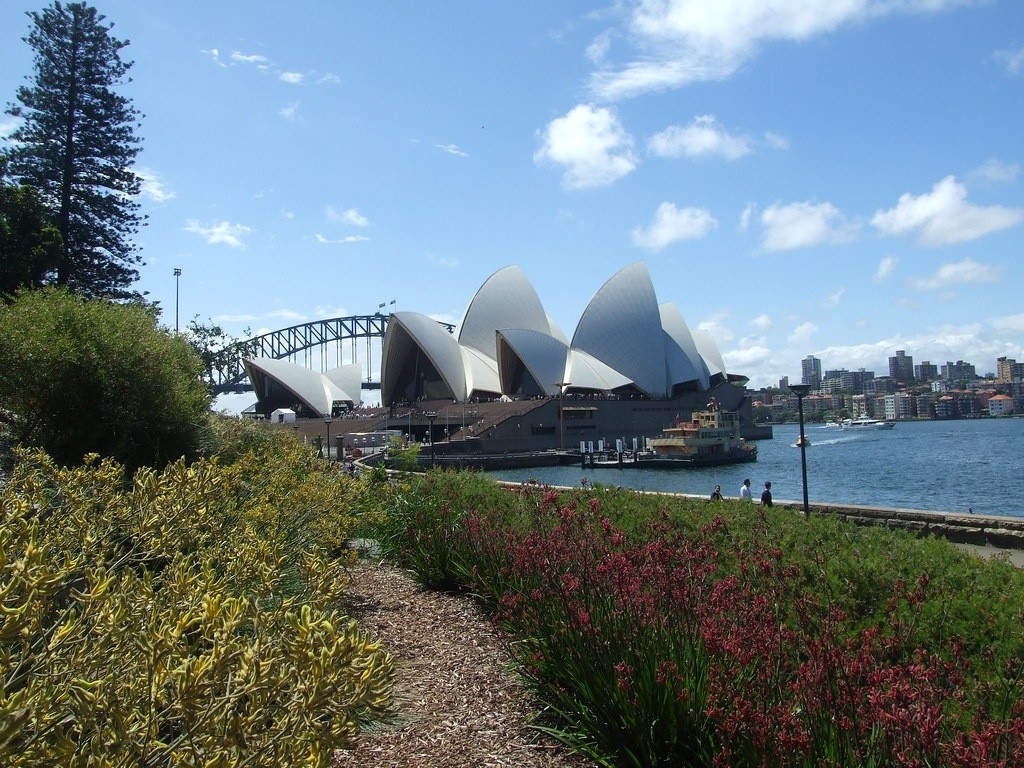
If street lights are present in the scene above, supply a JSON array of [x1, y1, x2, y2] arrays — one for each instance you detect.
[[172, 267, 182, 335], [324, 421, 333, 465], [426, 415, 439, 469], [555, 382, 572, 451], [786, 383, 813, 519]]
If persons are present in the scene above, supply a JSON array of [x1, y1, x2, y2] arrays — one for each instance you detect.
[[760, 481, 772, 507], [740, 479, 751, 502], [291, 392, 643, 478], [710, 485, 724, 500]]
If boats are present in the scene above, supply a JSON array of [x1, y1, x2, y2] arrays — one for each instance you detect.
[[646, 396, 758, 468], [795, 434, 810, 447], [826, 422, 839, 428], [842, 411, 897, 430]]
